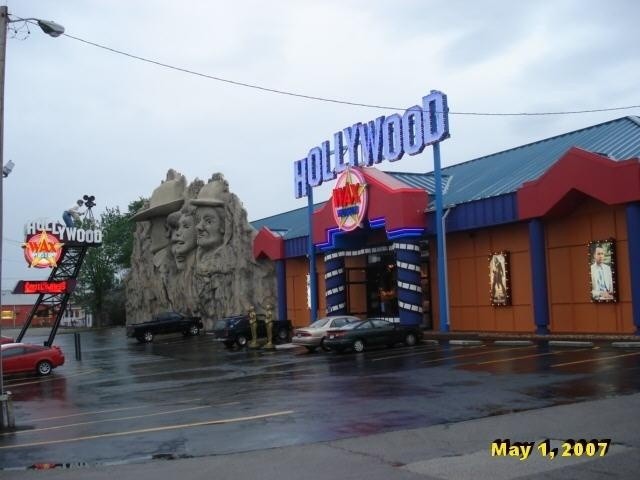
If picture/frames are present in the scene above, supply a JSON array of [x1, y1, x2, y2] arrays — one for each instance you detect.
[[587, 238, 619, 305], [488, 250, 512, 307], [306, 273, 318, 311]]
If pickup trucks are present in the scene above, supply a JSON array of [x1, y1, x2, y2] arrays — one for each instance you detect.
[[126, 311, 204, 344]]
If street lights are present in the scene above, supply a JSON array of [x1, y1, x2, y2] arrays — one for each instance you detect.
[[1, 5, 66, 189]]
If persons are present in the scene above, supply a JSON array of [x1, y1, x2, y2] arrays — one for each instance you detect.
[[127, 179, 236, 330], [264, 304, 273, 344], [249, 306, 257, 344], [591, 246, 613, 299], [492, 258, 506, 299], [63, 200, 85, 227]]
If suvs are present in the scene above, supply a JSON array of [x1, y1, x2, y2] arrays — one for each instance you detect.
[[212, 314, 293, 348]]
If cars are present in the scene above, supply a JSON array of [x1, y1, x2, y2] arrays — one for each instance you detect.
[[322, 317, 424, 353], [291, 316, 362, 352], [0, 335, 65, 377]]
